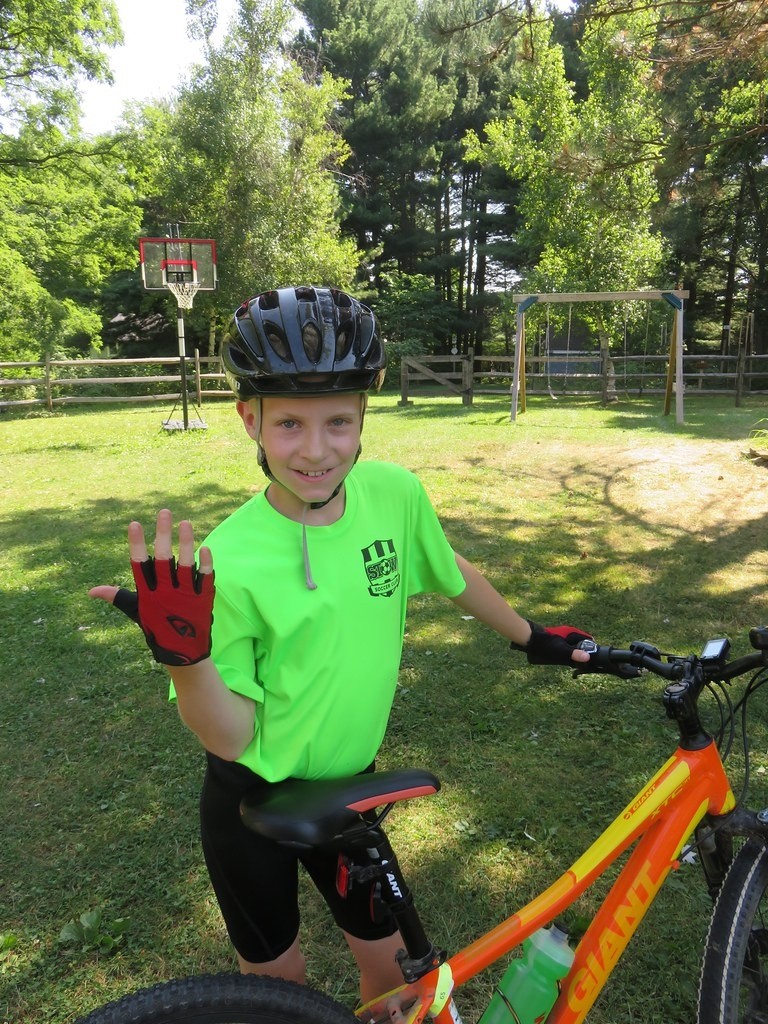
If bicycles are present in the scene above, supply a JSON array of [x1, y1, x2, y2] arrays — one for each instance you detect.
[[71, 625, 767, 1023]]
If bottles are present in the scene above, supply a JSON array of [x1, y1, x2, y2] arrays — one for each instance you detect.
[[475, 921, 575, 1024]]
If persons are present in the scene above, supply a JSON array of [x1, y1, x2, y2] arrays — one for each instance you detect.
[[89, 286, 602, 1024]]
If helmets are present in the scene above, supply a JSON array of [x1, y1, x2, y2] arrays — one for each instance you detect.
[[220, 287, 388, 403]]
